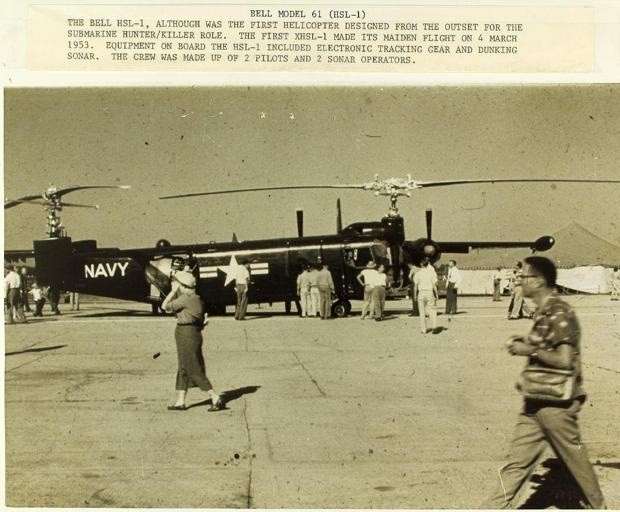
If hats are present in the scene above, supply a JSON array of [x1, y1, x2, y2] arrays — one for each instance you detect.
[[366, 261, 377, 268], [176, 270, 197, 288]]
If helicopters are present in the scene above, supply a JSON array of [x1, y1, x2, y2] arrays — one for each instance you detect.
[[4, 173, 620, 317]]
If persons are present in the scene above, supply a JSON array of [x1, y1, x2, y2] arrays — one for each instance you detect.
[[44, 286, 61, 316], [19, 266, 33, 313], [483, 255, 607, 510], [491, 268, 502, 301], [509, 260, 535, 319], [370, 263, 387, 322], [5, 264, 27, 324], [406, 262, 419, 316], [413, 260, 442, 334], [69, 291, 79, 311], [316, 264, 336, 320], [356, 261, 377, 319], [445, 259, 459, 314], [161, 270, 225, 412], [610, 267, 620, 300], [27, 283, 45, 317], [233, 259, 251, 320], [296, 265, 312, 319], [283, 300, 303, 315], [306, 265, 320, 318], [424, 256, 438, 282], [504, 268, 525, 318]]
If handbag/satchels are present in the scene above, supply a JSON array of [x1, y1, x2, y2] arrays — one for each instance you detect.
[[513, 363, 581, 405]]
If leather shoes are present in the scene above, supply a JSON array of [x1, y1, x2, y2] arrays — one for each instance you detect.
[[166, 397, 225, 411]]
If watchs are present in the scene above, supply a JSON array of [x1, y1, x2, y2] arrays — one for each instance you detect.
[[530, 348, 539, 361]]
